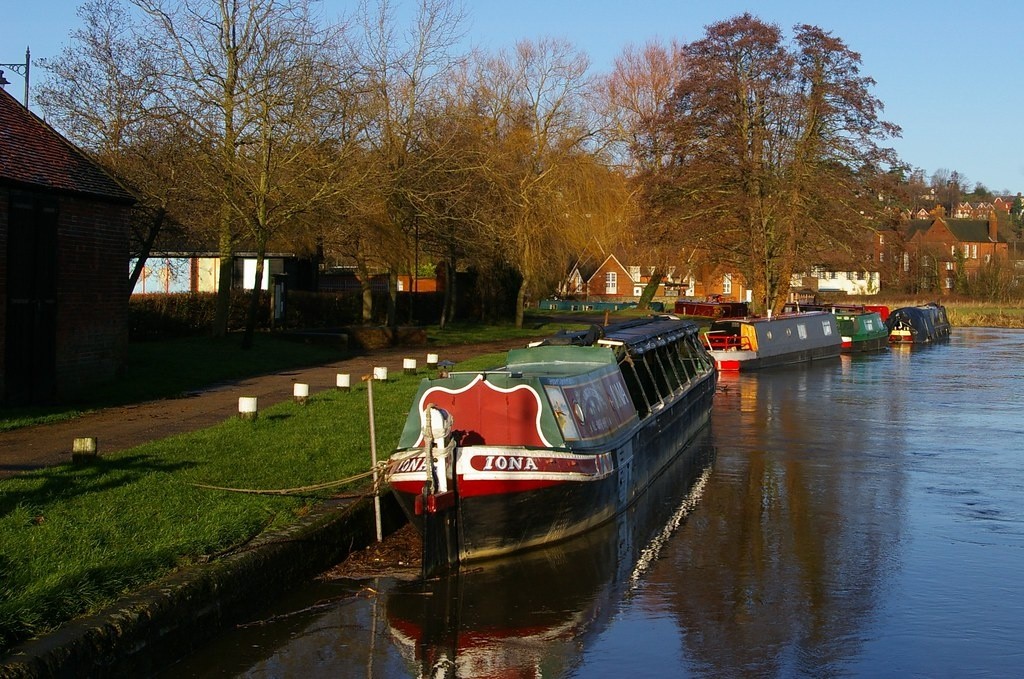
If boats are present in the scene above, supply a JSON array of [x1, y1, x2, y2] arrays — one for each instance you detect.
[[382, 313, 717, 510], [837, 311, 890, 349], [540, 300, 665, 313], [672, 293, 889, 333], [705, 310, 844, 373], [885, 302, 953, 345]]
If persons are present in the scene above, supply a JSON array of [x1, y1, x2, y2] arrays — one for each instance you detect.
[[708, 294, 736, 303], [796, 296, 829, 307], [556, 400, 568, 418], [760, 294, 776, 310]]
